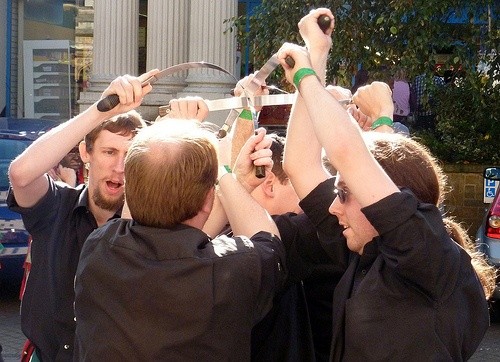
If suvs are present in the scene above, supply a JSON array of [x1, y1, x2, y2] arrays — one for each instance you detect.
[[0, 119, 63, 302]]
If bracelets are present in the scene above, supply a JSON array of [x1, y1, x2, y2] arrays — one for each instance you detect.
[[293, 67, 317, 90], [224, 164, 233, 173], [371, 116, 393, 130], [239, 109, 259, 121]]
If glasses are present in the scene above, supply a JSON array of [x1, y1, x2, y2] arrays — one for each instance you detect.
[[331, 185, 351, 204]]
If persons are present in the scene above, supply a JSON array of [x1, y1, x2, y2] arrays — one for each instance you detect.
[[392, 68, 443, 131], [6, 6, 498, 362]]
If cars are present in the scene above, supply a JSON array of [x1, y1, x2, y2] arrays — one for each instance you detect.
[[475, 167, 500, 321]]
